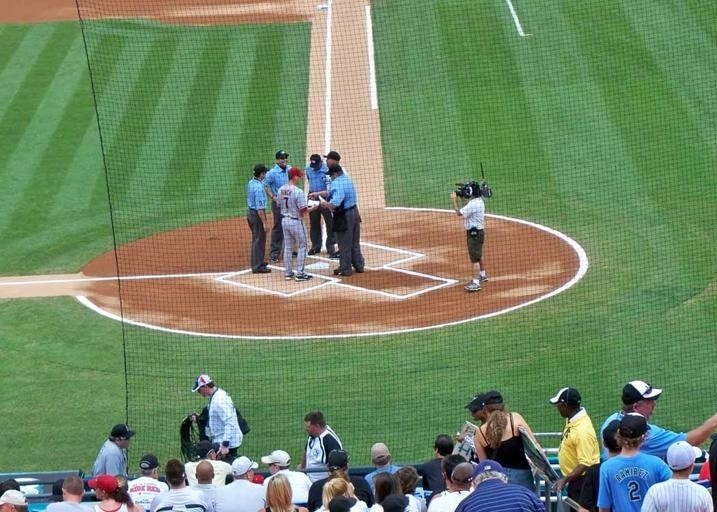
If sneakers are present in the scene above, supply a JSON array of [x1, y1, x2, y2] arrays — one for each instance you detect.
[[248, 247, 365, 281], [463, 275, 488, 291]]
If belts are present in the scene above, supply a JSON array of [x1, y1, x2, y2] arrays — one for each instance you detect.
[[343, 204, 356, 211]]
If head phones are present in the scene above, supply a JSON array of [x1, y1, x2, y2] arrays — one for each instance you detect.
[[465, 181, 474, 196]]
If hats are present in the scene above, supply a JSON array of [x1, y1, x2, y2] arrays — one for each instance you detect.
[[0, 489, 28, 506], [196, 440, 214, 458], [87, 476, 117, 492], [232, 456, 259, 476], [383, 496, 409, 512], [140, 455, 161, 470], [261, 449, 291, 467], [253, 151, 342, 180], [192, 375, 212, 392], [549, 387, 581, 404], [371, 443, 391, 460], [464, 392, 503, 409], [111, 424, 135, 440], [619, 412, 651, 438], [474, 460, 503, 477], [622, 380, 662, 405], [329, 496, 356, 512], [667, 441, 702, 470], [329, 449, 348, 468]]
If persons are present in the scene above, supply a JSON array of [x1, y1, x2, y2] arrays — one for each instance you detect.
[[0, 373, 717, 511], [322, 151, 347, 258], [246, 163, 271, 273], [277, 167, 318, 281], [451, 181, 488, 291], [264, 150, 298, 263], [307, 164, 364, 276], [306, 154, 337, 255]]
